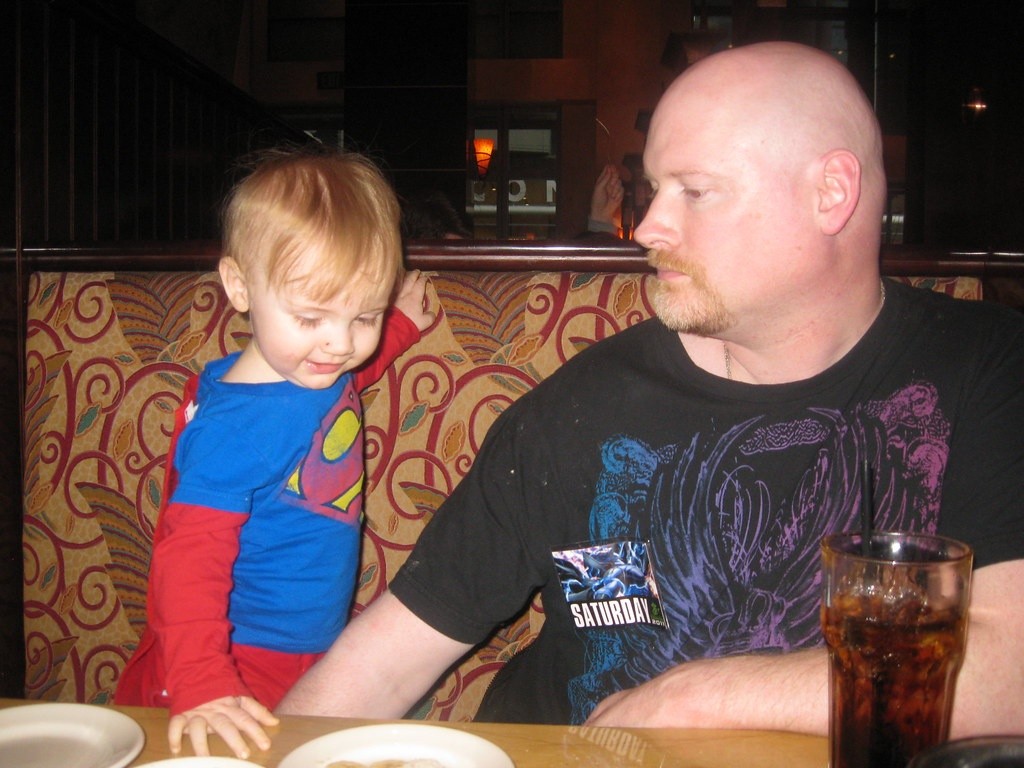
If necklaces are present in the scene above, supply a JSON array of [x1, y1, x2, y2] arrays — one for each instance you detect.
[[723, 280, 885, 380]]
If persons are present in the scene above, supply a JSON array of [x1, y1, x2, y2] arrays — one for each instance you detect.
[[113, 151, 435, 758], [276, 40, 1024, 738], [397, 164, 625, 239]]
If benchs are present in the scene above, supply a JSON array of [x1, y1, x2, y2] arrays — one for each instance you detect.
[[0, 243, 1024, 721]]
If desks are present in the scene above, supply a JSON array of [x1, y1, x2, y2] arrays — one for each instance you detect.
[[0, 698, 829, 768]]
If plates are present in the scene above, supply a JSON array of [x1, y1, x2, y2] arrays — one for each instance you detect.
[[133, 757, 268, 768], [0, 702, 145, 768], [278, 724, 515, 768]]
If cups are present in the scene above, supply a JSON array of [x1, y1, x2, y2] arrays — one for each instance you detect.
[[820, 531, 973, 768]]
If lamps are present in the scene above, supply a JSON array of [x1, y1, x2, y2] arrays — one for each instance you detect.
[[474, 138, 495, 181]]
